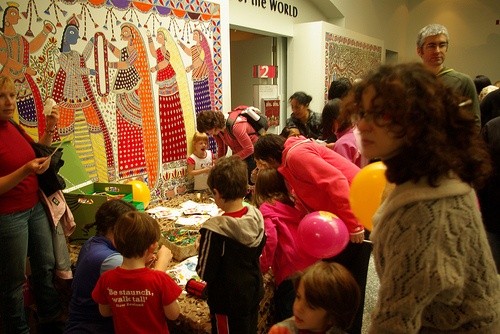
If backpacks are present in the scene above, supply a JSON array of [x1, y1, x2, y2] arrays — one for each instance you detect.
[[225, 105, 272, 138]]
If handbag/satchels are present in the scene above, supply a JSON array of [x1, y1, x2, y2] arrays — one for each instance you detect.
[[31, 142, 66, 196]]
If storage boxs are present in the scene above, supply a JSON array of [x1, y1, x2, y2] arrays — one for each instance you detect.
[[49, 138, 136, 250]]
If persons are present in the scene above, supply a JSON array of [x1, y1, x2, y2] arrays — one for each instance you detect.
[[196, 156, 267, 334], [268, 261, 361, 334], [417, 24, 481, 144], [185, 74, 500, 322], [354, 63, 500, 334], [68, 198, 173, 334], [0, 75, 67, 334], [91, 210, 180, 334]]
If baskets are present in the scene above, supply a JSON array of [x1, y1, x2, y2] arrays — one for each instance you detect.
[[161, 218, 200, 261]]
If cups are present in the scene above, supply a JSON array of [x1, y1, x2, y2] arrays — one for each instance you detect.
[[184, 278, 206, 297]]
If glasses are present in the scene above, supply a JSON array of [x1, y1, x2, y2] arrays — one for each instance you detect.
[[354, 102, 393, 125], [422, 43, 446, 51]]
